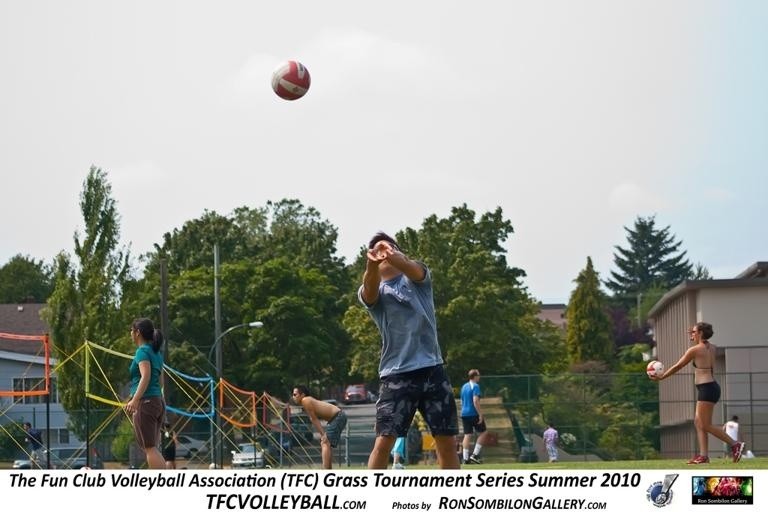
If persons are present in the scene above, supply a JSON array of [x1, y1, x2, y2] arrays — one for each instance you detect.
[[292, 384, 348, 469], [389, 432, 408, 468], [356, 229, 464, 469], [21, 420, 56, 469], [721, 415, 746, 459], [458, 369, 488, 465], [646, 319, 747, 466], [542, 421, 561, 463], [159, 423, 179, 469], [124, 316, 167, 469]]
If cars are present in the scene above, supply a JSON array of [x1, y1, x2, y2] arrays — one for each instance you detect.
[[173, 434, 209, 459], [345, 383, 372, 404], [230, 441, 267, 469]]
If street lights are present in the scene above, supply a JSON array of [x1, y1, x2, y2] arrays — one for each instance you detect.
[[209, 320, 263, 366]]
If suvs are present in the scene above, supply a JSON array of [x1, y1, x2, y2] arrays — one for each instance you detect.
[[11, 446, 103, 469]]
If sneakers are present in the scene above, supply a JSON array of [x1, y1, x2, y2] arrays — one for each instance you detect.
[[730, 440, 746, 462], [686, 454, 711, 465], [391, 453, 483, 469]]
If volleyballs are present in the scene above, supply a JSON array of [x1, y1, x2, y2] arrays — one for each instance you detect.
[[270, 61, 310, 100], [647, 360, 665, 377]]
